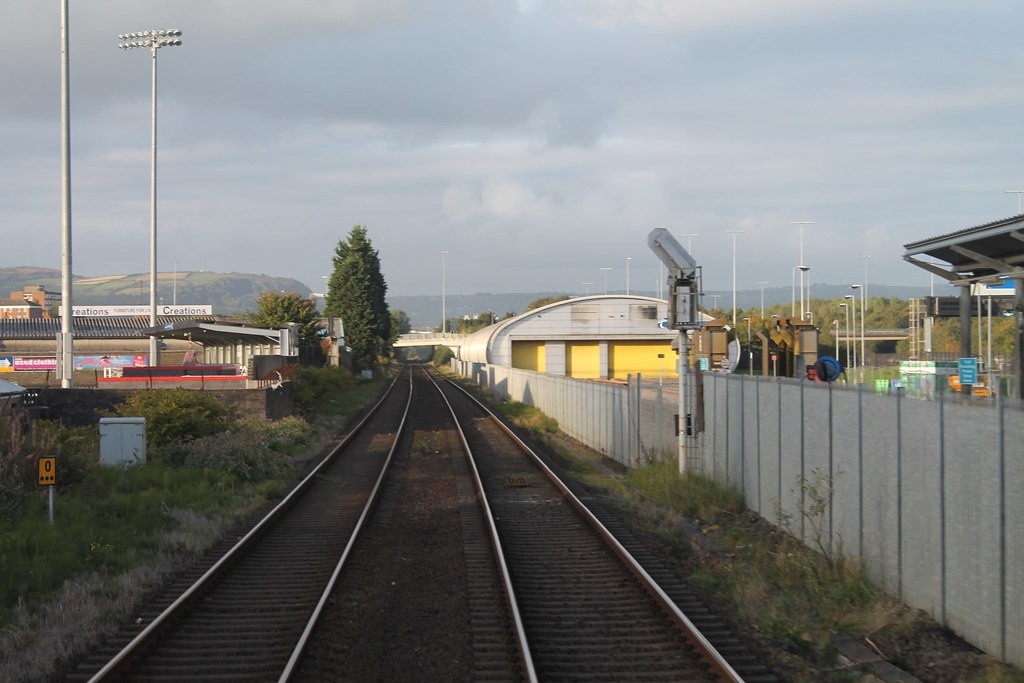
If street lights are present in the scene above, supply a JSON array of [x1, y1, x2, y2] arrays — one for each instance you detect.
[[599, 219, 818, 312], [434, 236, 453, 335], [834, 278, 884, 380], [117, 28, 183, 367]]
